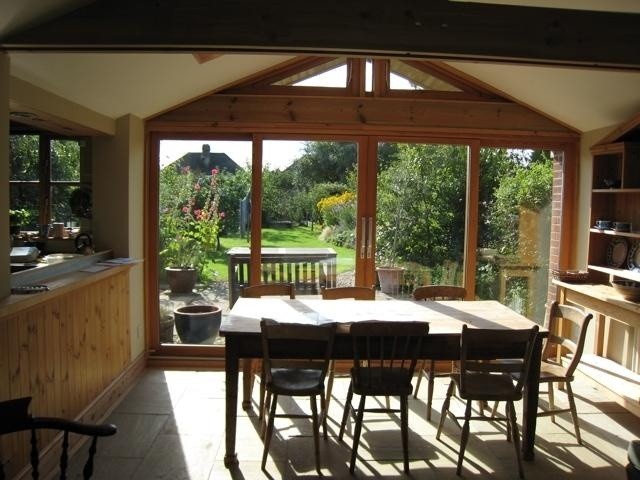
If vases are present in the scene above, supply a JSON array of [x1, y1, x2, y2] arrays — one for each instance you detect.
[[175, 305, 221, 347], [157, 308, 174, 344]]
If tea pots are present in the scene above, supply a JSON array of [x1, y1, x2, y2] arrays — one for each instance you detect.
[[75, 233, 97, 255]]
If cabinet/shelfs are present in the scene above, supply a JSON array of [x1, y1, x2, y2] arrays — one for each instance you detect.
[[551, 279, 640, 396], [587, 111, 640, 301]]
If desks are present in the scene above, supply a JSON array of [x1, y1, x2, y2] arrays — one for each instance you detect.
[[477, 255, 540, 322]]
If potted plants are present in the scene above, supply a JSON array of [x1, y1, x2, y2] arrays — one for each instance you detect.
[[158, 225, 200, 293], [9, 209, 31, 235], [376, 195, 407, 295]]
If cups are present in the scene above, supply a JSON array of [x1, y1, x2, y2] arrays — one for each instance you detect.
[[38, 226, 50, 238], [597, 221, 612, 228], [53, 223, 64, 239], [614, 222, 628, 230]]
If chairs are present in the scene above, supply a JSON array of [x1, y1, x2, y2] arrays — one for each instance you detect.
[[626, 439, 640, 480], [490, 299, 593, 450], [241, 284, 314, 408], [338, 321, 429, 476], [1, 417, 118, 480], [320, 285, 377, 399], [260, 318, 337, 473], [436, 324, 539, 479], [412, 285, 489, 422]]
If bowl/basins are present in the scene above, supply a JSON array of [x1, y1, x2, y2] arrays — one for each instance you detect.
[[610, 280, 640, 302]]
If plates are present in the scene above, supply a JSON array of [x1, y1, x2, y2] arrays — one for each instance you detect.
[[594, 226, 611, 231], [627, 240, 639, 270], [606, 238, 627, 269], [612, 228, 630, 233]]
